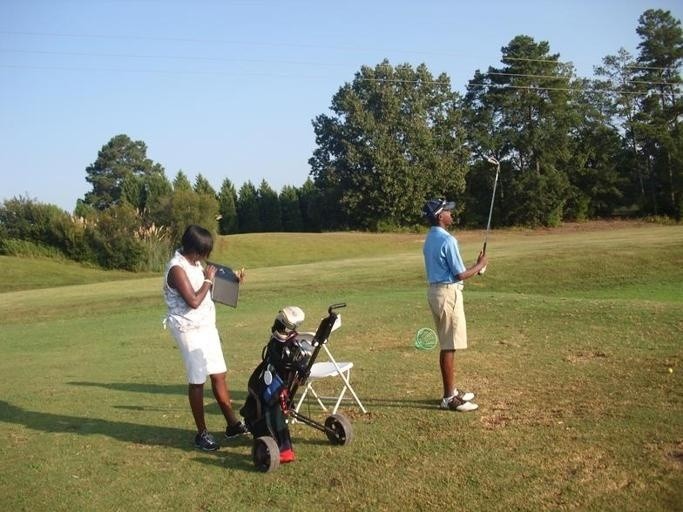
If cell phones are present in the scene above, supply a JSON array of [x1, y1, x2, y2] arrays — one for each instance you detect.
[[481, 154, 499, 165]]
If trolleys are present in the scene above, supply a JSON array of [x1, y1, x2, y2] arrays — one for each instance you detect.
[[236, 303, 354, 475]]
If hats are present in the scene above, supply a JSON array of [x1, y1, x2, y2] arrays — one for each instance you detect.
[[423, 198, 455, 217]]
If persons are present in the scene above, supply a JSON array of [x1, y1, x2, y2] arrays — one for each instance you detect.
[[418, 197, 489, 412], [159, 224, 252, 453]]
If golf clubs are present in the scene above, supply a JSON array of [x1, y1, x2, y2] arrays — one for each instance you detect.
[[281, 339, 311, 360], [478, 153, 500, 275]]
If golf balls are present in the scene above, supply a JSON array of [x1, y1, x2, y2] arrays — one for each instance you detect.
[[667, 367, 674, 373]]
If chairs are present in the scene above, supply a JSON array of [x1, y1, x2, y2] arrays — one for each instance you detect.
[[293, 333, 372, 414]]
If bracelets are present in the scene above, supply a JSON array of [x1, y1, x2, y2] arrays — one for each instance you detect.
[[203, 279, 212, 284]]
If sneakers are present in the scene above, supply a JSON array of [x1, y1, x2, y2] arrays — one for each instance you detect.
[[440, 396, 478, 412], [453, 387, 474, 401], [194, 431, 220, 451], [225, 422, 251, 439]]
